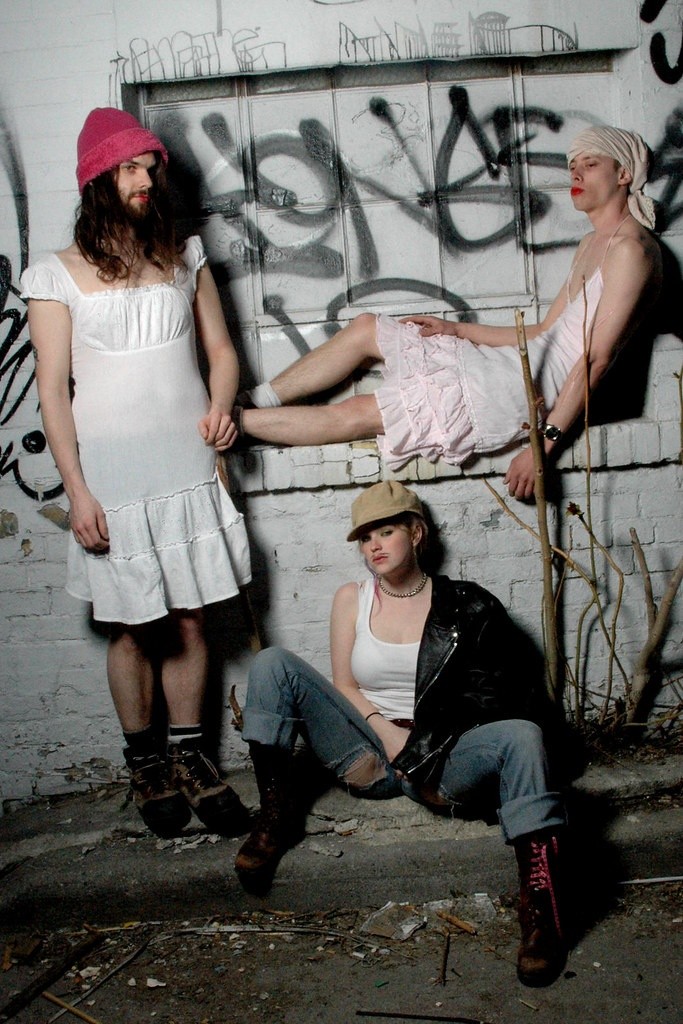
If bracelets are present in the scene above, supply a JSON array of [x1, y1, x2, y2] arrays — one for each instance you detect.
[[364, 712, 383, 719]]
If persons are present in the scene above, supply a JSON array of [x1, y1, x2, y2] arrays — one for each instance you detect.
[[236, 481, 587, 988], [20, 107, 250, 839], [232, 126, 662, 500]]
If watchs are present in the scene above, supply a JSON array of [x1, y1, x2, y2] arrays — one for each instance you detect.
[[543, 423, 561, 442]]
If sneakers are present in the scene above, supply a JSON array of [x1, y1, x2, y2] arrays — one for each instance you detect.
[[165, 736, 241, 828], [123, 745, 192, 836]]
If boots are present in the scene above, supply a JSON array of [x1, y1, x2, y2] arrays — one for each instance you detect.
[[235, 745, 305, 875], [514, 827, 568, 987]]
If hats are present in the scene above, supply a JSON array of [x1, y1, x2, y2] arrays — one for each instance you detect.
[[76, 107, 167, 196], [347, 480, 423, 542]]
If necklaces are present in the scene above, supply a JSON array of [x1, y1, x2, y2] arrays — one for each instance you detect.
[[379, 574, 427, 596]]
